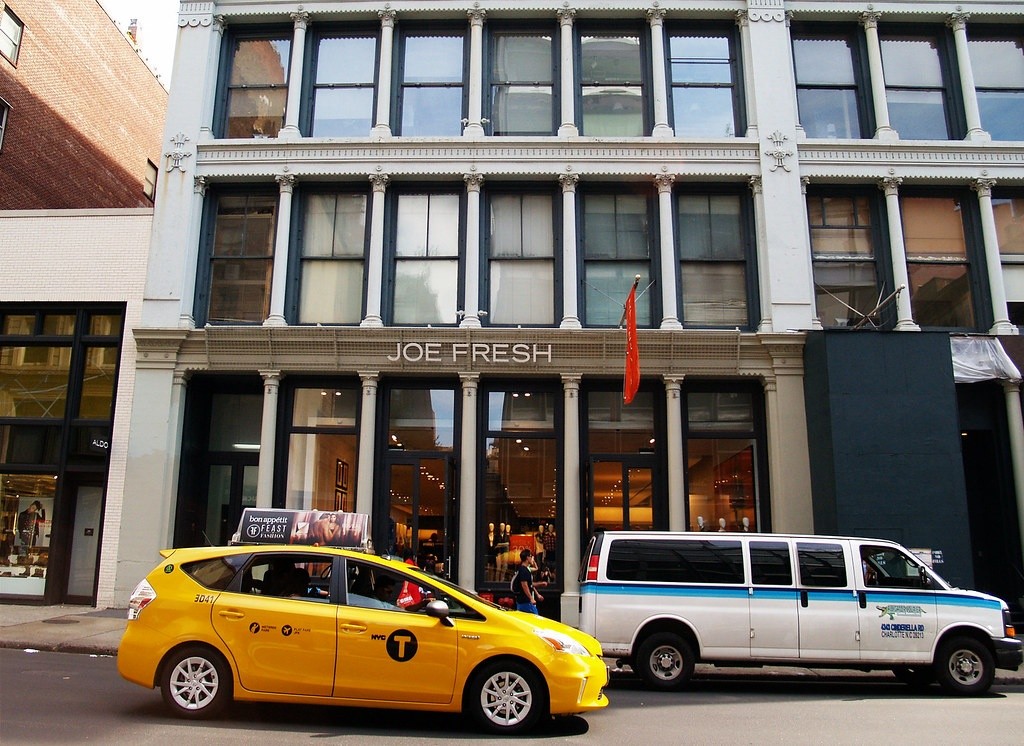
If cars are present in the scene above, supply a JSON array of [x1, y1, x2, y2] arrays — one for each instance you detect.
[[115, 540, 610, 737]]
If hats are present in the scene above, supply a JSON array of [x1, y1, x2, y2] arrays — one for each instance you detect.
[[34, 501, 41, 511]]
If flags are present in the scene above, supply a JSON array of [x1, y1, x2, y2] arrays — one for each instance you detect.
[[623, 284, 640, 405]]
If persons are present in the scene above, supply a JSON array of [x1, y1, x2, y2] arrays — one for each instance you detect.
[[697, 516, 750, 532], [535, 524, 556, 583], [397, 548, 439, 611], [374, 576, 396, 603], [487, 523, 511, 582], [510, 549, 548, 615], [291, 568, 328, 597], [19, 500, 45, 546], [313, 513, 340, 545]]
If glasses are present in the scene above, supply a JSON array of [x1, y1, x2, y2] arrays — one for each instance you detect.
[[528, 555, 532, 558]]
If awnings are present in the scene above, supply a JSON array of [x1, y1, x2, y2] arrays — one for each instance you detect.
[[948, 335, 1021, 382]]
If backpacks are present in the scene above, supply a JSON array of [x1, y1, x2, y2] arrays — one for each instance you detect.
[[510, 571, 521, 594]]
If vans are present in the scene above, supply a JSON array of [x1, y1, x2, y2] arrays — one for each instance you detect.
[[575, 529, 1024, 698]]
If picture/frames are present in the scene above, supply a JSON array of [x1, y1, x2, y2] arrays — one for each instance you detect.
[[334, 458, 349, 512]]
